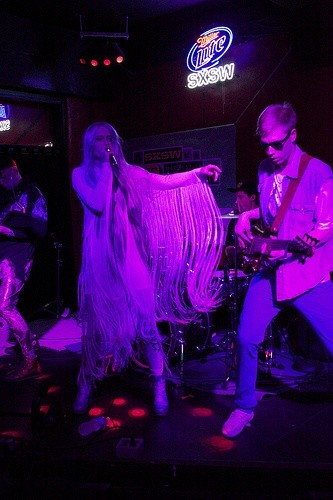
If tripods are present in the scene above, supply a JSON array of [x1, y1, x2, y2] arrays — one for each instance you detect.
[[202, 219, 241, 389], [27, 244, 82, 324]]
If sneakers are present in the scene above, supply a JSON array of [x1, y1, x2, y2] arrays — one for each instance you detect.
[[222, 409, 255, 438], [4, 359, 40, 382]]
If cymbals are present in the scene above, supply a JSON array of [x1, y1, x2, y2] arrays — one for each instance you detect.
[[215, 210, 240, 219]]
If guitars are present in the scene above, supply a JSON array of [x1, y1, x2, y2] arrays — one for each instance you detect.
[[240, 225, 314, 275]]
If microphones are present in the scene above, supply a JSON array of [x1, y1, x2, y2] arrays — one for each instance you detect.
[[105, 148, 118, 169], [51, 233, 59, 245]]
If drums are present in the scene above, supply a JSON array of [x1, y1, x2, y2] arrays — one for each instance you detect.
[[156, 321, 191, 367], [188, 311, 227, 353], [212, 268, 250, 326]]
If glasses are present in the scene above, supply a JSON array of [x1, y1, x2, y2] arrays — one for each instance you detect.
[[259, 132, 291, 149]]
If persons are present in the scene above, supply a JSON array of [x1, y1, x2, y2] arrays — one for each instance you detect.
[[72, 103, 333, 438], [0, 159, 48, 379]]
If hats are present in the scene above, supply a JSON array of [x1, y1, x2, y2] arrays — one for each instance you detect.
[[227, 179, 258, 192]]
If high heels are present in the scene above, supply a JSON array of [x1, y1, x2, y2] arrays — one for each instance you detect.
[[152, 375, 169, 417], [72, 374, 93, 414]]
